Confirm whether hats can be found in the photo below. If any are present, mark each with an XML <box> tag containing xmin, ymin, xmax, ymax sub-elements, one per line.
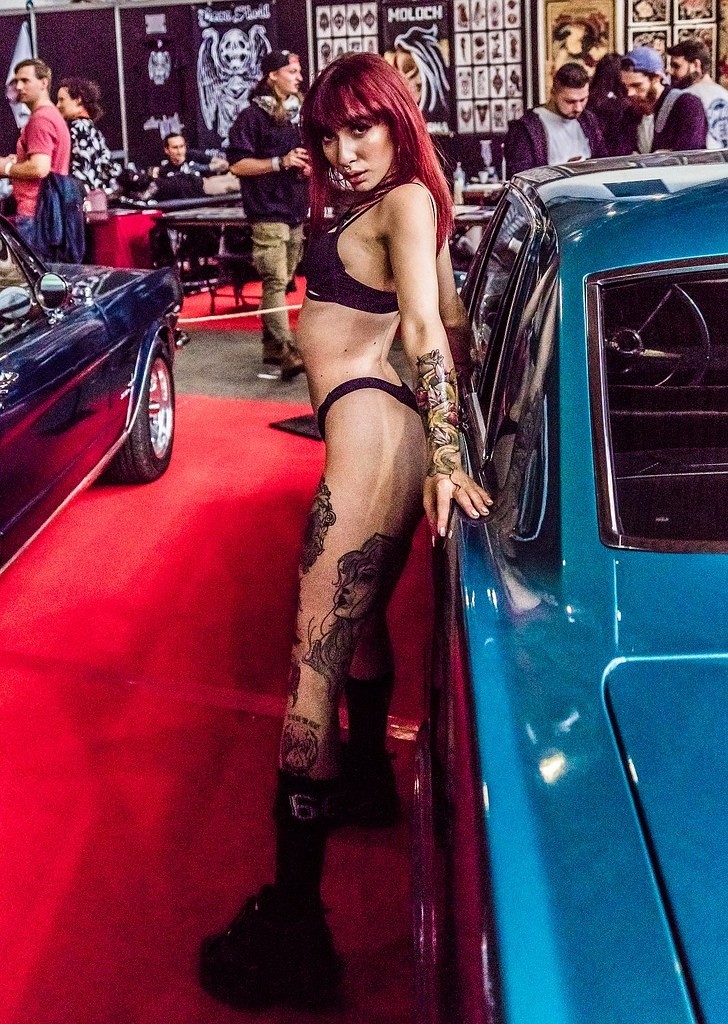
<box><xmin>261</xmin><ymin>49</ymin><xmax>299</xmax><ymax>72</ymax></box>
<box><xmin>621</xmin><ymin>46</ymin><xmax>668</xmax><ymax>80</ymax></box>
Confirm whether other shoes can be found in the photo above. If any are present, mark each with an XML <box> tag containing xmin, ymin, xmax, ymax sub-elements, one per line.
<box><xmin>262</xmin><ymin>336</ymin><xmax>283</xmax><ymax>365</ymax></box>
<box><xmin>282</xmin><ymin>349</ymin><xmax>305</xmax><ymax>382</ymax></box>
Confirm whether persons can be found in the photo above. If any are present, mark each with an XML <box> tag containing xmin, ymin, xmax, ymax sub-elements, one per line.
<box><xmin>196</xmin><ymin>48</ymin><xmax>497</xmax><ymax>1020</ymax></box>
<box><xmin>55</xmin><ymin>76</ymin><xmax>119</xmax><ymax>266</ymax></box>
<box><xmin>586</xmin><ymin>51</ymin><xmax>635</xmax><ymax>125</ymax></box>
<box><xmin>605</xmin><ymin>45</ymin><xmax>710</xmax><ymax>157</ymax></box>
<box><xmin>224</xmin><ymin>50</ymin><xmax>312</xmax><ymax>382</ymax></box>
<box><xmin>502</xmin><ymin>62</ymin><xmax>611</xmax><ymax>183</ymax></box>
<box><xmin>0</xmin><ymin>58</ymin><xmax>72</xmax><ymax>262</ymax></box>
<box><xmin>134</xmin><ymin>134</ymin><xmax>230</xmax><ymax>297</ymax></box>
<box><xmin>664</xmin><ymin>40</ymin><xmax>727</xmax><ymax>149</ymax></box>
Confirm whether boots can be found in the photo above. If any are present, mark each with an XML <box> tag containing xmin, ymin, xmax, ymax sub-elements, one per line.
<box><xmin>203</xmin><ymin>770</ymin><xmax>334</xmax><ymax>1000</ymax></box>
<box><xmin>326</xmin><ymin>679</ymin><xmax>399</xmax><ymax>826</ymax></box>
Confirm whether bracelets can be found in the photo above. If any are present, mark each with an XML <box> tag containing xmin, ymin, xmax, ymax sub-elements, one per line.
<box><xmin>271</xmin><ymin>156</ymin><xmax>280</xmax><ymax>172</ymax></box>
<box><xmin>279</xmin><ymin>157</ymin><xmax>287</xmax><ymax>171</ymax></box>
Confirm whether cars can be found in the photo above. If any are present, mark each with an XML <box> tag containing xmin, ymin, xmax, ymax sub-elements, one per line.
<box><xmin>419</xmin><ymin>151</ymin><xmax>725</xmax><ymax>1024</ymax></box>
<box><xmin>0</xmin><ymin>215</ymin><xmax>186</xmax><ymax>574</ymax></box>
